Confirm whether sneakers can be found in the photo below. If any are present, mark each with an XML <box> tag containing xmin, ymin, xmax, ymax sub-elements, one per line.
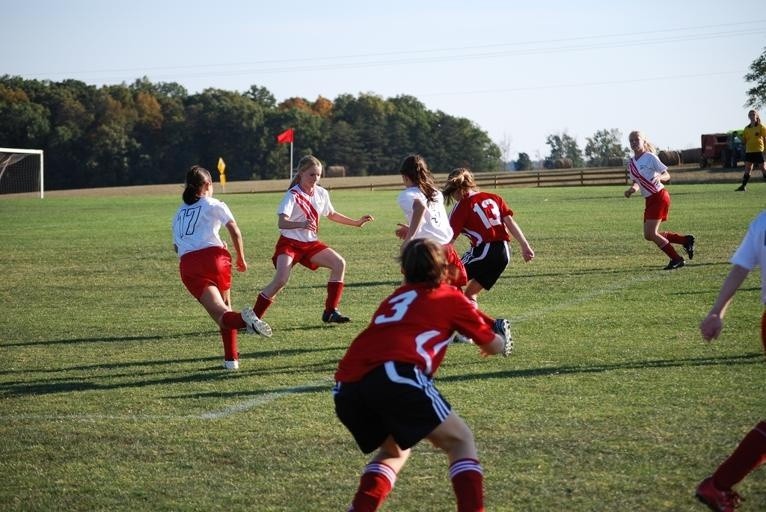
<box><xmin>223</xmin><ymin>359</ymin><xmax>240</xmax><ymax>371</ymax></box>
<box><xmin>320</xmin><ymin>308</ymin><xmax>353</xmax><ymax>324</ymax></box>
<box><xmin>240</xmin><ymin>306</ymin><xmax>273</xmax><ymax>339</ymax></box>
<box><xmin>663</xmin><ymin>257</ymin><xmax>685</xmax><ymax>270</ymax></box>
<box><xmin>682</xmin><ymin>234</ymin><xmax>695</xmax><ymax>261</ymax></box>
<box><xmin>692</xmin><ymin>476</ymin><xmax>744</xmax><ymax>512</ymax></box>
<box><xmin>492</xmin><ymin>317</ymin><xmax>515</xmax><ymax>358</ymax></box>
<box><xmin>733</xmin><ymin>184</ymin><xmax>747</xmax><ymax>193</ymax></box>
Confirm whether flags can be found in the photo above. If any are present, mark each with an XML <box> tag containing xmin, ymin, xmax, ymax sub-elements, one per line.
<box><xmin>278</xmin><ymin>129</ymin><xmax>293</xmax><ymax>143</ymax></box>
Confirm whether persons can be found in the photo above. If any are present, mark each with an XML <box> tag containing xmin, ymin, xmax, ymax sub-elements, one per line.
<box><xmin>734</xmin><ymin>110</ymin><xmax>766</xmax><ymax>191</ymax></box>
<box><xmin>395</xmin><ymin>155</ymin><xmax>513</xmax><ymax>357</ymax></box>
<box><xmin>331</xmin><ymin>238</ymin><xmax>504</xmax><ymax>512</ymax></box>
<box><xmin>172</xmin><ymin>165</ymin><xmax>272</xmax><ymax>370</ymax></box>
<box><xmin>625</xmin><ymin>131</ymin><xmax>695</xmax><ymax>270</ymax></box>
<box><xmin>438</xmin><ymin>167</ymin><xmax>535</xmax><ymax>343</ymax></box>
<box><xmin>699</xmin><ymin>211</ymin><xmax>766</xmax><ymax>511</ymax></box>
<box><xmin>246</xmin><ymin>155</ymin><xmax>375</xmax><ymax>333</ymax></box>
